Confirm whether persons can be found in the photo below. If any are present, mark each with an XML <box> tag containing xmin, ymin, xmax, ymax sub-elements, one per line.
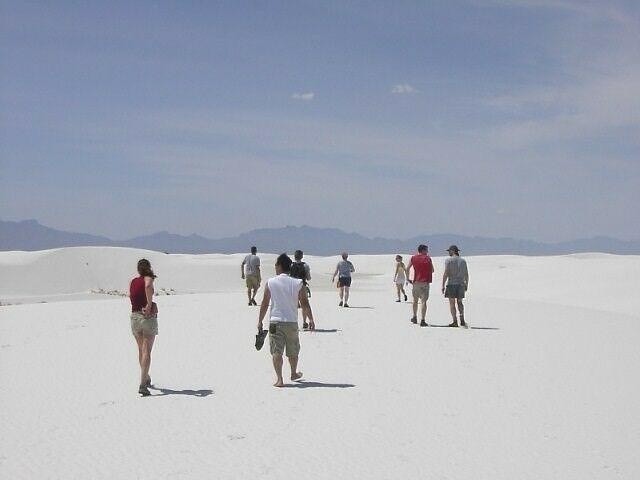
<box><xmin>258</xmin><ymin>255</ymin><xmax>315</xmax><ymax>386</ymax></box>
<box><xmin>290</xmin><ymin>250</ymin><xmax>311</xmax><ymax>329</ymax></box>
<box><xmin>405</xmin><ymin>245</ymin><xmax>434</xmax><ymax>327</ymax></box>
<box><xmin>241</xmin><ymin>246</ymin><xmax>262</xmax><ymax>306</ymax></box>
<box><xmin>393</xmin><ymin>255</ymin><xmax>407</xmax><ymax>302</ymax></box>
<box><xmin>442</xmin><ymin>245</ymin><xmax>469</xmax><ymax>328</ymax></box>
<box><xmin>333</xmin><ymin>253</ymin><xmax>354</xmax><ymax>307</ymax></box>
<box><xmin>129</xmin><ymin>259</ymin><xmax>159</xmax><ymax>395</ymax></box>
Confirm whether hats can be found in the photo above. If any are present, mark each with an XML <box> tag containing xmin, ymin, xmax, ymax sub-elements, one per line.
<box><xmin>446</xmin><ymin>245</ymin><xmax>458</xmax><ymax>251</ymax></box>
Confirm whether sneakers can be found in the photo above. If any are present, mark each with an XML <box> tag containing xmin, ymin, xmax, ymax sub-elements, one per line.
<box><xmin>449</xmin><ymin>320</ymin><xmax>465</xmax><ymax>326</ymax></box>
<box><xmin>339</xmin><ymin>301</ymin><xmax>349</xmax><ymax>307</ymax></box>
<box><xmin>411</xmin><ymin>316</ymin><xmax>428</xmax><ymax>326</ymax></box>
<box><xmin>139</xmin><ymin>380</ymin><xmax>150</xmax><ymax>395</ymax></box>
<box><xmin>396</xmin><ymin>295</ymin><xmax>407</xmax><ymax>302</ymax></box>
<box><xmin>255</xmin><ymin>328</ymin><xmax>267</xmax><ymax>350</ymax></box>
<box><xmin>303</xmin><ymin>323</ymin><xmax>308</xmax><ymax>328</ymax></box>
<box><xmin>249</xmin><ymin>298</ymin><xmax>256</xmax><ymax>306</ymax></box>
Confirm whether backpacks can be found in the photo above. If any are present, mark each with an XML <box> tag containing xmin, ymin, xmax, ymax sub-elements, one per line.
<box><xmin>290</xmin><ymin>262</ymin><xmax>305</xmax><ymax>286</ymax></box>
